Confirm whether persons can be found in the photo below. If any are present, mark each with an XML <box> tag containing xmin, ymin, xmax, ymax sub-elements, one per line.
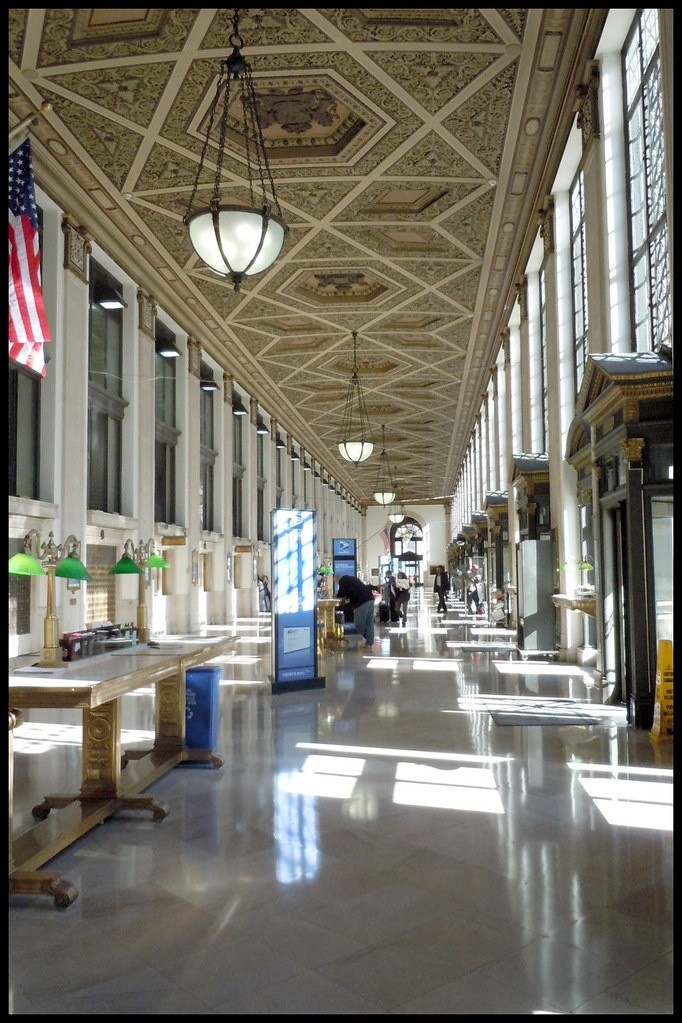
<box><xmin>334</xmin><ymin>574</ymin><xmax>375</xmax><ymax>648</ymax></box>
<box><xmin>318</xmin><ymin>573</ymin><xmax>325</xmax><ymax>598</ymax></box>
<box><xmin>257</xmin><ymin>574</ymin><xmax>271</xmax><ymax>612</ymax></box>
<box><xmin>434</xmin><ymin>565</ymin><xmax>450</xmax><ymax>613</ymax></box>
<box><xmin>469</xmin><ymin>565</ymin><xmax>479</xmax><ymax>614</ymax></box>
<box><xmin>377</xmin><ymin>570</ymin><xmax>410</xmax><ymax>625</ymax></box>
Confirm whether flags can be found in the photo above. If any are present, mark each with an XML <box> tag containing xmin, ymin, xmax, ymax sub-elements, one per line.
<box><xmin>8</xmin><ymin>136</ymin><xmax>52</xmax><ymax>380</ymax></box>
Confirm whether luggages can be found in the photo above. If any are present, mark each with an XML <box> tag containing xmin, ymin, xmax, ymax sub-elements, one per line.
<box><xmin>379</xmin><ymin>587</ymin><xmax>389</xmax><ymax>623</ymax></box>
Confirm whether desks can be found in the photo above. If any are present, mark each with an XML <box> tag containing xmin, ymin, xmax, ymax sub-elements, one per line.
<box><xmin>552</xmin><ymin>593</ymin><xmax>596</xmax><ymax>619</ymax></box>
<box><xmin>9</xmin><ymin>636</ymin><xmax>239</xmax><ymax>908</ymax></box>
<box><xmin>317</xmin><ymin>597</ymin><xmax>351</xmax><ymax>679</ymax></box>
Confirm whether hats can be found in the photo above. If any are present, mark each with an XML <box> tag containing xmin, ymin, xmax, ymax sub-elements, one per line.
<box><xmin>472</xmin><ymin>564</ymin><xmax>478</xmax><ymax>570</ymax></box>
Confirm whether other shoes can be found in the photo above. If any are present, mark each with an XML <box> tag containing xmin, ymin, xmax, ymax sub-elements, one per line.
<box><xmin>359</xmin><ymin>644</ymin><xmax>373</xmax><ymax>648</ymax></box>
<box><xmin>402</xmin><ymin>622</ymin><xmax>405</xmax><ymax>627</ymax></box>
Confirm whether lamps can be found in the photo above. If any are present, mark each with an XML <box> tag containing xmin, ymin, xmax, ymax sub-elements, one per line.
<box><xmin>155</xmin><ymin>336</ymin><xmax>182</xmax><ymax>360</ymax></box>
<box><xmin>201</xmin><ymin>374</ymin><xmax>220</xmax><ymax>393</ymax></box>
<box><xmin>106</xmin><ymin>537</ymin><xmax>172</xmax><ymax>645</ymax></box>
<box><xmin>317</xmin><ymin>561</ymin><xmax>334</xmax><ymax>600</ymax></box>
<box><xmin>574</xmin><ymin>555</ymin><xmax>596</xmax><ymax>598</ymax></box>
<box><xmin>181</xmin><ymin>9</ymin><xmax>290</xmax><ymax>293</ymax></box>
<box><xmin>373</xmin><ymin>424</ymin><xmax>397</xmax><ymax>510</ymax></box>
<box><xmin>397</xmin><ymin>504</ymin><xmax>413</xmax><ymax>542</ymax></box>
<box><xmin>336</xmin><ymin>331</ymin><xmax>376</xmax><ymax>469</ymax></box>
<box><xmin>8</xmin><ymin>530</ymin><xmax>94</xmax><ymax>670</ymax></box>
<box><xmin>386</xmin><ymin>486</ymin><xmax>405</xmax><ymax>524</ymax></box>
<box><xmin>233</xmin><ymin>399</ymin><xmax>368</xmax><ymax>519</ymax></box>
<box><xmin>93</xmin><ymin>273</ymin><xmax>127</xmax><ymax>311</ymax></box>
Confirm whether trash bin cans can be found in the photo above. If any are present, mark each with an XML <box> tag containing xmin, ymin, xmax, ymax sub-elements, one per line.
<box><xmin>186</xmin><ymin>665</ymin><xmax>223</xmax><ymax>750</ymax></box>
